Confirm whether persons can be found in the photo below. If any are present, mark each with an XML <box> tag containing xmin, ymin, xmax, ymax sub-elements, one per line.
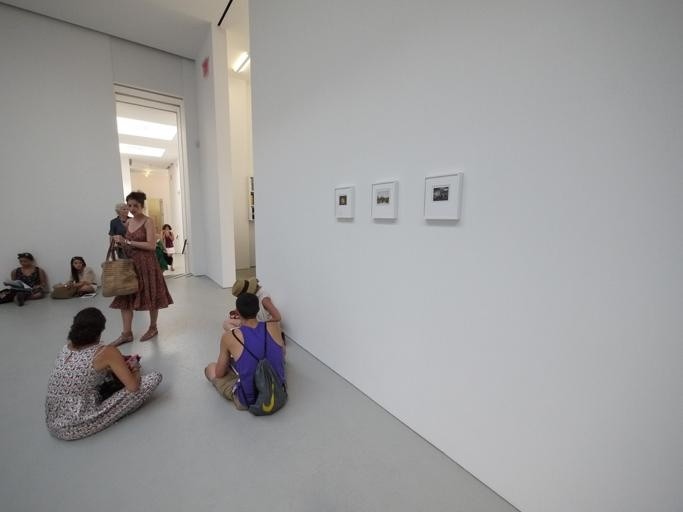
<box><xmin>204</xmin><ymin>293</ymin><xmax>287</xmax><ymax>411</ymax></box>
<box><xmin>222</xmin><ymin>277</ymin><xmax>281</xmax><ymax>332</ymax></box>
<box><xmin>66</xmin><ymin>256</ymin><xmax>97</xmax><ymax>296</ymax></box>
<box><xmin>161</xmin><ymin>224</ymin><xmax>174</xmax><ymax>271</ymax></box>
<box><xmin>109</xmin><ymin>203</ymin><xmax>133</xmax><ymax>257</ymax></box>
<box><xmin>0</xmin><ymin>253</ymin><xmax>48</xmax><ymax>306</ymax></box>
<box><xmin>45</xmin><ymin>307</ymin><xmax>163</xmax><ymax>442</ymax></box>
<box><xmin>106</xmin><ymin>190</ymin><xmax>174</xmax><ymax>348</ymax></box>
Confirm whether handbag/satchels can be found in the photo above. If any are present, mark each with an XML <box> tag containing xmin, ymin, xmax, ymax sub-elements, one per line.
<box><xmin>51</xmin><ymin>283</ymin><xmax>78</xmax><ymax>298</ymax></box>
<box><xmin>101</xmin><ymin>238</ymin><xmax>139</xmax><ymax>297</ymax></box>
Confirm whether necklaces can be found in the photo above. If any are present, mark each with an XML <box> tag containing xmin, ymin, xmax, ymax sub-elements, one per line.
<box><xmin>120</xmin><ymin>218</ymin><xmax>128</xmax><ymax>223</ymax></box>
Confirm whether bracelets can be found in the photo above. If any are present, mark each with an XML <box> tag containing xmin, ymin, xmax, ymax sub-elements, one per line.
<box><xmin>127</xmin><ymin>240</ymin><xmax>131</xmax><ymax>246</ymax></box>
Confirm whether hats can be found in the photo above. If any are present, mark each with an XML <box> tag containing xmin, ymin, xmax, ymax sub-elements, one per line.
<box><xmin>232</xmin><ymin>277</ymin><xmax>258</xmax><ymax>297</ymax></box>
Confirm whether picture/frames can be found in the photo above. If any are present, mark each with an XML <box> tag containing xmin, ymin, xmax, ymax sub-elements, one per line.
<box><xmin>424</xmin><ymin>172</ymin><xmax>463</xmax><ymax>220</ymax></box>
<box><xmin>371</xmin><ymin>181</ymin><xmax>397</xmax><ymax>219</ymax></box>
<box><xmin>334</xmin><ymin>186</ymin><xmax>355</xmax><ymax>218</ymax></box>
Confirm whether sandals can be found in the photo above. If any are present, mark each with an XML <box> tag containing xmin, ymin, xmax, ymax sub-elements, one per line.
<box><xmin>140</xmin><ymin>326</ymin><xmax>158</xmax><ymax>341</ymax></box>
<box><xmin>112</xmin><ymin>332</ymin><xmax>133</xmax><ymax>346</ymax></box>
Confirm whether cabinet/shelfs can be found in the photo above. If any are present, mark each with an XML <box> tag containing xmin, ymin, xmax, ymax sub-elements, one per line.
<box><xmin>247</xmin><ymin>177</ymin><xmax>256</xmax><ymax>267</ymax></box>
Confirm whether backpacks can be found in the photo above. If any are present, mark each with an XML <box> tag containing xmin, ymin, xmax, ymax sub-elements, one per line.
<box><xmin>232</xmin><ymin>322</ymin><xmax>287</xmax><ymax>415</ymax></box>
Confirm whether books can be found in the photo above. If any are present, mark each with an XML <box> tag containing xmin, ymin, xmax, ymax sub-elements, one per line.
<box><xmin>2</xmin><ymin>279</ymin><xmax>32</xmax><ymax>290</ymax></box>
